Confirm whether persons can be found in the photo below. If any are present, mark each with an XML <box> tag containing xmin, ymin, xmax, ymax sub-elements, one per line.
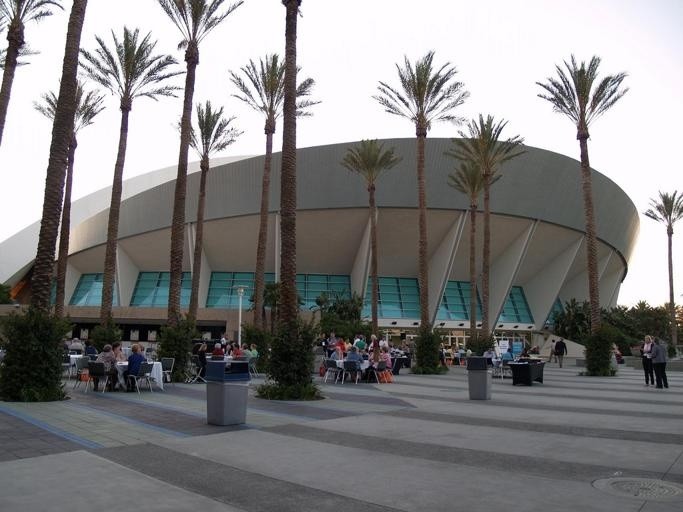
<box><xmin>554</xmin><ymin>337</ymin><xmax>567</xmax><ymax>368</ymax></box>
<box><xmin>646</xmin><ymin>337</ymin><xmax>668</xmax><ymax>388</ymax></box>
<box><xmin>190</xmin><ymin>335</ymin><xmax>257</xmax><ymax>382</ymax></box>
<box><xmin>481</xmin><ymin>347</ymin><xmax>496</xmax><ymax>364</ymax></box>
<box><xmin>501</xmin><ymin>348</ymin><xmax>515</xmax><ymax>362</ymax></box>
<box><xmin>640</xmin><ymin>335</ymin><xmax>654</xmax><ymax>385</ymax></box>
<box><xmin>314</xmin><ymin>328</ymin><xmax>472</xmax><ymax>384</ymax></box>
<box><xmin>546</xmin><ymin>339</ymin><xmax>557</xmax><ymax>363</ymax></box>
<box><xmin>60</xmin><ymin>337</ymin><xmax>147</xmax><ymax>392</ymax></box>
<box><xmin>517</xmin><ymin>345</ymin><xmax>540</xmax><ymax>363</ymax></box>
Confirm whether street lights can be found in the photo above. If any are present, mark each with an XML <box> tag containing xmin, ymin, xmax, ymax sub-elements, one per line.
<box><xmin>231</xmin><ymin>285</ymin><xmax>248</xmax><ymax>348</ymax></box>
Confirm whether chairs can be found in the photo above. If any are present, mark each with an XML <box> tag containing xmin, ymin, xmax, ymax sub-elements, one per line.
<box><xmin>59</xmin><ymin>343</ymin><xmax>175</xmax><ymax>394</ymax></box>
<box><xmin>442</xmin><ymin>350</ymin><xmax>538</xmax><ymax>384</ymax></box>
<box><xmin>183</xmin><ymin>351</ymin><xmax>260</xmax><ymax>384</ymax></box>
<box><xmin>321</xmin><ymin>352</ymin><xmax>413</xmax><ymax>386</ymax></box>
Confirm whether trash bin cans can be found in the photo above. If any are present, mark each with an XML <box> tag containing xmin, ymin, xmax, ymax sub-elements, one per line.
<box><xmin>466</xmin><ymin>356</ymin><xmax>493</xmax><ymax>399</ymax></box>
<box><xmin>206</xmin><ymin>361</ymin><xmax>252</xmax><ymax>426</ymax></box>
